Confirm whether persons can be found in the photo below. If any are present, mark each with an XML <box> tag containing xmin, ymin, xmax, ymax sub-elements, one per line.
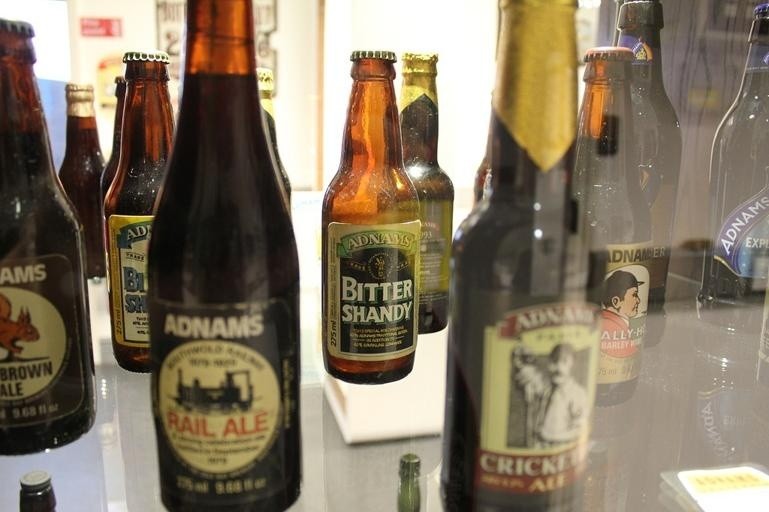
<box><xmin>598</xmin><ymin>270</ymin><xmax>646</xmax><ymax>339</ymax></box>
<box><xmin>514</xmin><ymin>343</ymin><xmax>586</xmax><ymax>450</ymax></box>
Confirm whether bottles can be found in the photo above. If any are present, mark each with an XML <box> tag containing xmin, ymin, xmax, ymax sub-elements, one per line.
<box><xmin>390</xmin><ymin>51</ymin><xmax>455</xmax><ymax>338</ymax></box>
<box><xmin>18</xmin><ymin>470</ymin><xmax>58</xmax><ymax>512</ymax></box>
<box><xmin>396</xmin><ymin>453</ymin><xmax>422</xmax><ymax>511</ymax></box>
<box><xmin>611</xmin><ymin>1</ymin><xmax>686</xmax><ymax>348</ymax></box>
<box><xmin>435</xmin><ymin>1</ymin><xmax>604</xmax><ymax>511</ymax></box>
<box><xmin>315</xmin><ymin>50</ymin><xmax>424</xmax><ymax>390</ymax></box>
<box><xmin>144</xmin><ymin>2</ymin><xmax>300</xmax><ymax>511</ymax></box>
<box><xmin>98</xmin><ymin>42</ymin><xmax>178</xmax><ymax>379</ymax></box>
<box><xmin>54</xmin><ymin>82</ymin><xmax>111</xmax><ymax>286</ymax></box>
<box><xmin>695</xmin><ymin>4</ymin><xmax>769</xmax><ymax>334</ymax></box>
<box><xmin>0</xmin><ymin>16</ymin><xmax>96</xmax><ymax>459</ymax></box>
<box><xmin>575</xmin><ymin>48</ymin><xmax>646</xmax><ymax>404</ymax></box>
